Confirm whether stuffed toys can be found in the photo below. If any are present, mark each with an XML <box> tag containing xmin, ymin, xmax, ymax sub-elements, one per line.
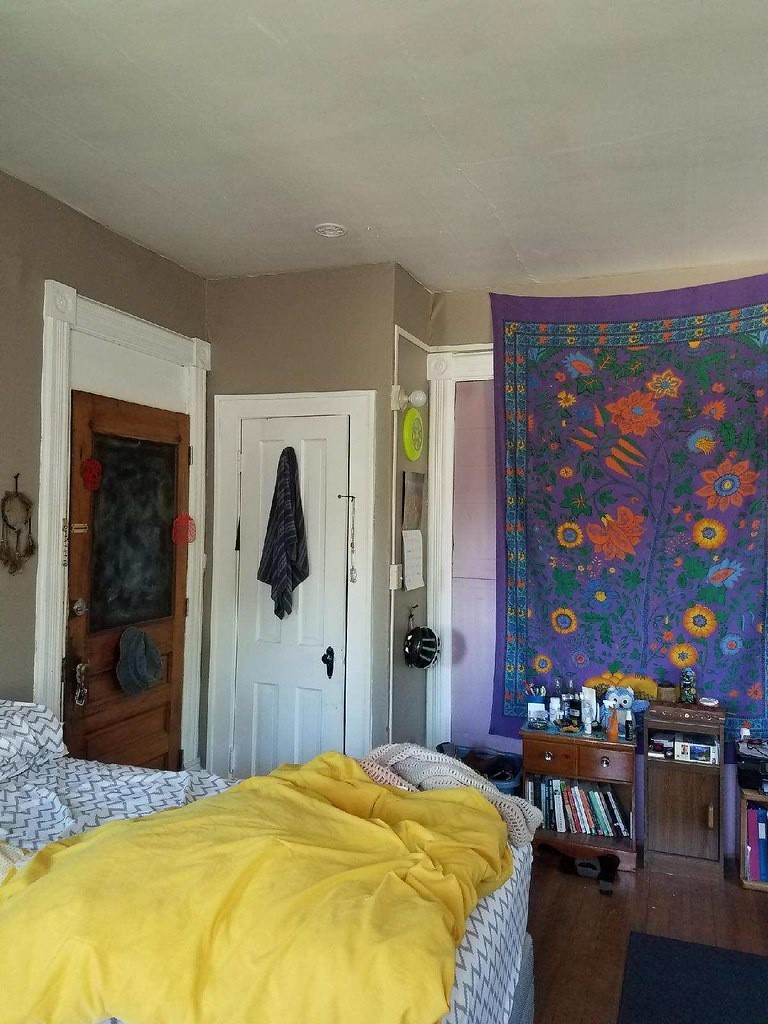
<box><xmin>598</xmin><ymin>681</ymin><xmax>650</xmax><ymax>738</ymax></box>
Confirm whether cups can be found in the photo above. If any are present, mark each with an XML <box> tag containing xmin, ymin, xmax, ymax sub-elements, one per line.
<box><xmin>526</xmin><ymin>695</ymin><xmax>545</xmax><ymax>707</ymax></box>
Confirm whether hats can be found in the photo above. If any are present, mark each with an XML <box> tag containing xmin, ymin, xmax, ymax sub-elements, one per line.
<box><xmin>116</xmin><ymin>626</ymin><xmax>162</xmax><ymax>696</ymax></box>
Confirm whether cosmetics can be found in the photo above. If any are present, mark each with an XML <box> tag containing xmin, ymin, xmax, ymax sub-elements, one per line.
<box><xmin>520</xmin><ymin>679</ymin><xmax>633</xmax><ymax>743</ymax></box>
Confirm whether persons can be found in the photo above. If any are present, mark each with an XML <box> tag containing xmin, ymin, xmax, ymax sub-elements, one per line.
<box><xmin>680</xmin><ymin>744</ymin><xmax>688</xmax><ymax>754</ymax></box>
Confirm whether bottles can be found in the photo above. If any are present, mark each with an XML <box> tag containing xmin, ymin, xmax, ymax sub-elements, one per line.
<box><xmin>625</xmin><ymin>710</ymin><xmax>633</xmax><ymax>741</ymax></box>
<box><xmin>528</xmin><ymin>710</ymin><xmax>548</xmax><ymax>730</ymax></box>
<box><xmin>548</xmin><ymin>677</ymin><xmax>585</xmax><ymax>727</ymax></box>
<box><xmin>680</xmin><ymin>667</ymin><xmax>696</xmax><ymax>703</ymax></box>
<box><xmin>658</xmin><ymin>682</ymin><xmax>676</xmax><ymax>702</ymax></box>
<box><xmin>739</xmin><ymin>728</ymin><xmax>751</xmax><ymax>740</ymax></box>
<box><xmin>584</xmin><ymin>717</ymin><xmax>592</xmax><ymax>734</ymax></box>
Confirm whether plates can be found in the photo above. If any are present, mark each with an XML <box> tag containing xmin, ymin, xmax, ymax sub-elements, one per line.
<box><xmin>700</xmin><ymin>696</ymin><xmax>719</xmax><ymax>707</ymax></box>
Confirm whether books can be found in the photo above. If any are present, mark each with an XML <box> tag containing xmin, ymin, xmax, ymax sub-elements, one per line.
<box><xmin>746</xmin><ymin>808</ymin><xmax>767</xmax><ymax>883</ymax></box>
<box><xmin>524</xmin><ymin>774</ymin><xmax>629</xmax><ymax>838</ymax></box>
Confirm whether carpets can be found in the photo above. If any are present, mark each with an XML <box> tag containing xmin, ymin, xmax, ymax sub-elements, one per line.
<box><xmin>616</xmin><ymin>931</ymin><xmax>768</xmax><ymax>1024</ymax></box>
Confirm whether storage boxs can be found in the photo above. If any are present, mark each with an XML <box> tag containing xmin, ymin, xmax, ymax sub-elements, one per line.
<box><xmin>435</xmin><ymin>741</ymin><xmax>523</xmax><ymax>795</ymax></box>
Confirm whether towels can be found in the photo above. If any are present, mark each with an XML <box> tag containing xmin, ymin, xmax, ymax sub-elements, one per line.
<box><xmin>256</xmin><ymin>446</ymin><xmax>308</xmax><ymax>621</ymax></box>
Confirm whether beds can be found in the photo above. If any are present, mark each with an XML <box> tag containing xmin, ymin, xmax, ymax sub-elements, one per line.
<box><xmin>0</xmin><ymin>758</ymin><xmax>539</xmax><ymax>1024</ymax></box>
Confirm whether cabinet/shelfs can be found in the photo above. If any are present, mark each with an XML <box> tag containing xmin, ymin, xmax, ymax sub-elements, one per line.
<box><xmin>517</xmin><ymin>723</ymin><xmax>639</xmax><ymax>873</ymax></box>
<box><xmin>643</xmin><ymin>707</ymin><xmax>725</xmax><ymax>881</ymax></box>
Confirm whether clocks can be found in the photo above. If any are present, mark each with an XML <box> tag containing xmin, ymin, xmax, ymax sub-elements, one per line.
<box><xmin>402</xmin><ymin>408</ymin><xmax>424</xmax><ymax>462</ymax></box>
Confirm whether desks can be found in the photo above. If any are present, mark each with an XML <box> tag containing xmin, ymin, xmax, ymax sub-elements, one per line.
<box><xmin>736</xmin><ymin>788</ymin><xmax>768</xmax><ymax>893</ymax></box>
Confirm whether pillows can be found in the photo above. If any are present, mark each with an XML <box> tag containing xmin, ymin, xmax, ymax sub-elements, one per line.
<box><xmin>0</xmin><ymin>699</ymin><xmax>70</xmax><ymax>782</ymax></box>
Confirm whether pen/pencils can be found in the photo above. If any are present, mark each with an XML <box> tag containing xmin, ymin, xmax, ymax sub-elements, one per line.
<box><xmin>522</xmin><ymin>680</ymin><xmax>547</xmax><ymax>697</ymax></box>
